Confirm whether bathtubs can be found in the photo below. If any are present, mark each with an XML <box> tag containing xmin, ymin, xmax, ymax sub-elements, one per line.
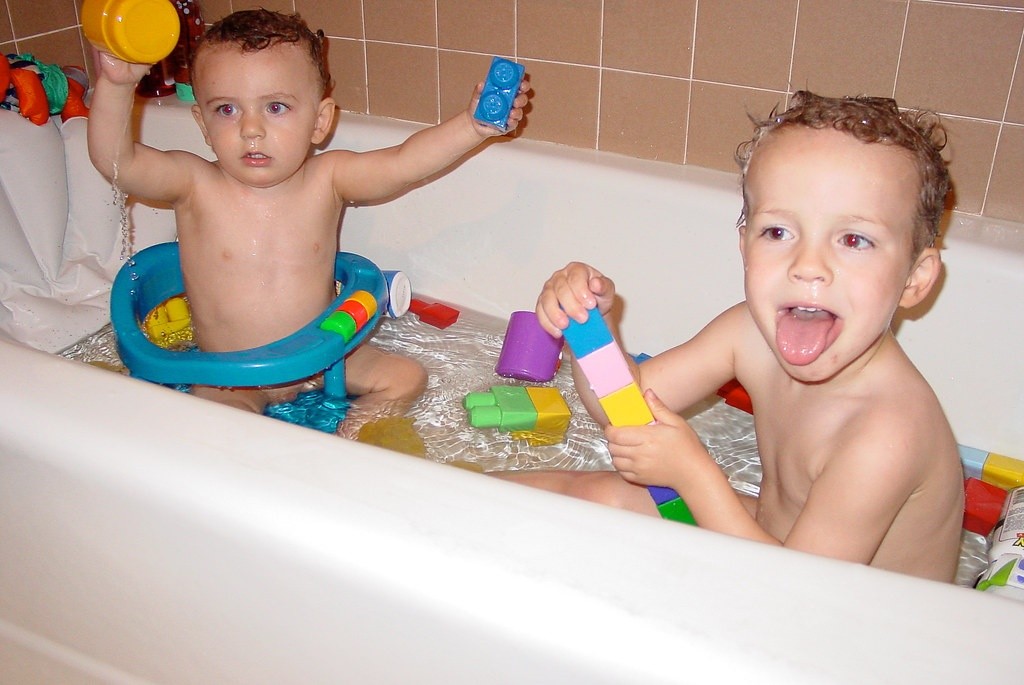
<box><xmin>0</xmin><ymin>88</ymin><xmax>1024</xmax><ymax>683</ymax></box>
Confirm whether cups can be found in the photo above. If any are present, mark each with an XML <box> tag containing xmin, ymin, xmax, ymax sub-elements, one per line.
<box><xmin>81</xmin><ymin>0</ymin><xmax>180</xmax><ymax>64</ymax></box>
<box><xmin>334</xmin><ymin>270</ymin><xmax>411</xmax><ymax>320</ymax></box>
<box><xmin>495</xmin><ymin>311</ymin><xmax>564</xmax><ymax>382</ymax></box>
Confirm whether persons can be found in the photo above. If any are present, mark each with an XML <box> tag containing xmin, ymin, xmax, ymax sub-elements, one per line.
<box><xmin>485</xmin><ymin>90</ymin><xmax>965</xmax><ymax>585</ymax></box>
<box><xmin>86</xmin><ymin>10</ymin><xmax>531</xmax><ymax>417</ymax></box>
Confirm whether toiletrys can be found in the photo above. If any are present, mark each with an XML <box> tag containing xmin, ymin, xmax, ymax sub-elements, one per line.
<box><xmin>135</xmin><ymin>1</ymin><xmax>207</xmax><ymax>102</ymax></box>
